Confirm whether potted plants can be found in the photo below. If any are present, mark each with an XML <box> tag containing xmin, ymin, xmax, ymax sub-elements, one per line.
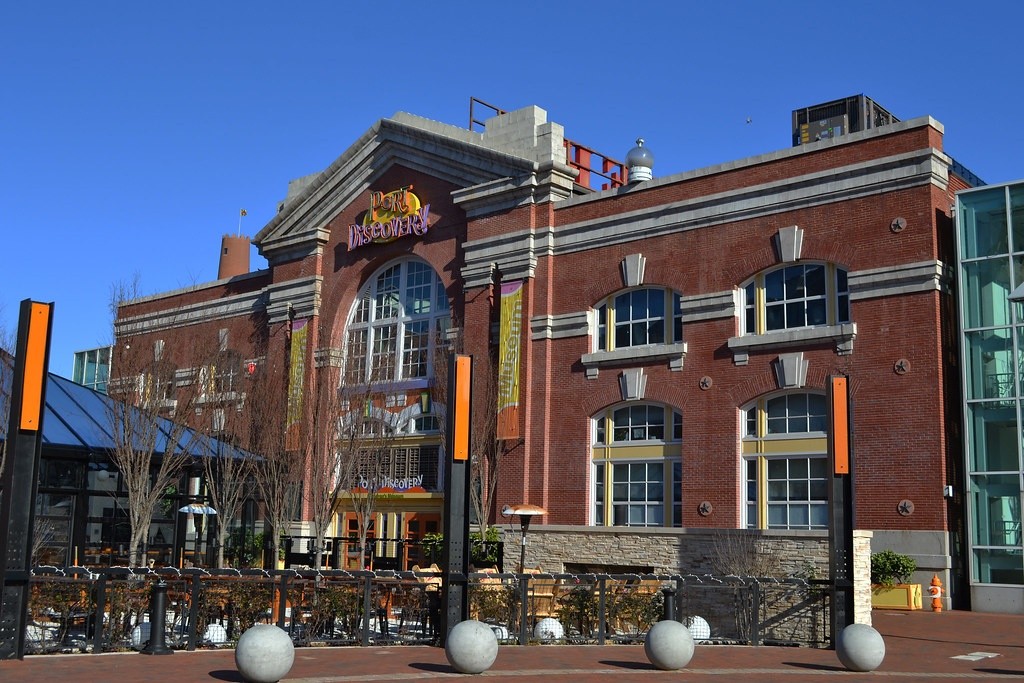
<box><xmin>871</xmin><ymin>548</ymin><xmax>923</xmax><ymax>611</ymax></box>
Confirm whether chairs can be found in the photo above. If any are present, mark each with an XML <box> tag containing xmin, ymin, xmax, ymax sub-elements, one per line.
<box><xmin>27</xmin><ymin>545</ymin><xmax>673</xmax><ymax>648</ymax></box>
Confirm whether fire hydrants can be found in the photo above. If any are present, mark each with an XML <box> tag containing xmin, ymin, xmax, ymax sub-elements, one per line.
<box><xmin>926</xmin><ymin>574</ymin><xmax>945</xmax><ymax>613</ymax></box>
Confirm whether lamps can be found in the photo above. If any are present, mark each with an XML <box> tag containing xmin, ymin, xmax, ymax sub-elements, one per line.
<box><xmin>98</xmin><ymin>470</ymin><xmax>119</xmax><ymax>483</ymax></box>
